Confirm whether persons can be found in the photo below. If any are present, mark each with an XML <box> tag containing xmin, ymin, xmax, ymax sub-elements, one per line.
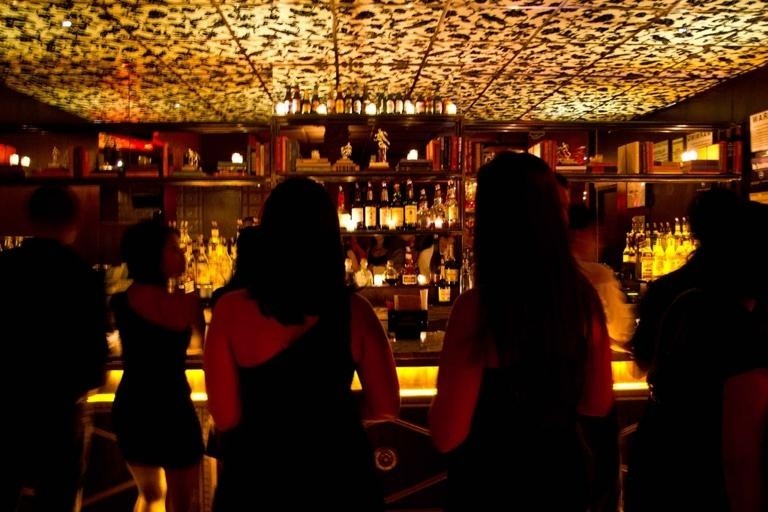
<box><xmin>0</xmin><ymin>180</ymin><xmax>110</xmax><ymax>510</ymax></box>
<box><xmin>422</xmin><ymin>148</ymin><xmax>618</xmax><ymax>511</ymax></box>
<box><xmin>549</xmin><ymin>170</ymin><xmax>573</xmax><ymax>230</ymax></box>
<box><xmin>102</xmin><ymin>213</ymin><xmax>206</xmax><ymax>509</ymax></box>
<box><xmin>210</xmin><ymin>224</ymin><xmax>262</xmax><ymax>310</ymax></box>
<box><xmin>200</xmin><ymin>172</ymin><xmax>404</xmax><ymax>510</ymax></box>
<box><xmin>626</xmin><ymin>185</ymin><xmax>768</xmax><ymax>509</ymax></box>
<box><xmin>561</xmin><ymin>200</ymin><xmax>638</xmax><ymax>350</ymax></box>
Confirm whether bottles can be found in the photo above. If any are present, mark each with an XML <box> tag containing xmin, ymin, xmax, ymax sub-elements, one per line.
<box><xmin>621</xmin><ymin>215</ymin><xmax>700</xmax><ymax>294</ymax></box>
<box><xmin>280</xmin><ymin>87</ymin><xmax>460</xmax><ymax>117</ymax></box>
<box><xmin>333</xmin><ymin>179</ymin><xmax>462</xmax><ymax>313</ymax></box>
<box><xmin>102</xmin><ymin>216</ymin><xmax>260</xmax><ymax>298</ymax></box>
